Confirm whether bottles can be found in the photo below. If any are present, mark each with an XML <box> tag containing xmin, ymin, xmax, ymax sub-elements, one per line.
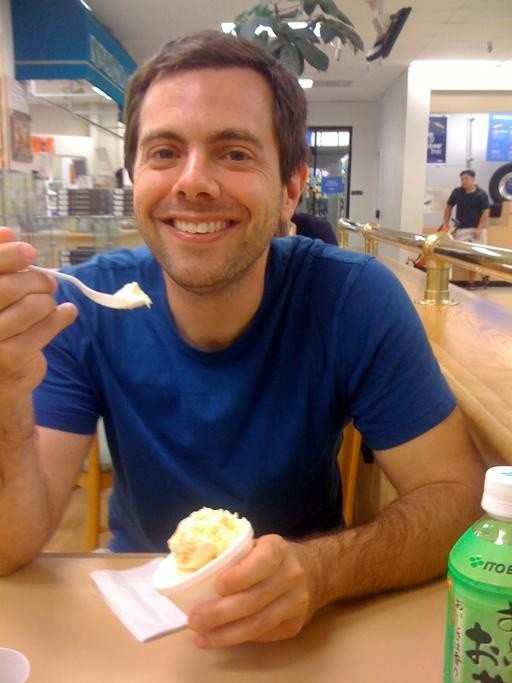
<box><xmin>445</xmin><ymin>465</ymin><xmax>511</xmax><ymax>682</ymax></box>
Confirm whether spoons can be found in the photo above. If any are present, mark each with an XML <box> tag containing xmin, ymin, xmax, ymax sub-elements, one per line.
<box><xmin>25</xmin><ymin>264</ymin><xmax>147</xmax><ymax>311</ymax></box>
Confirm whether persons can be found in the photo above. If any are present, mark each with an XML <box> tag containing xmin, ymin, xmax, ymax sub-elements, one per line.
<box><xmin>287</xmin><ymin>191</ymin><xmax>338</xmax><ymax>246</ymax></box>
<box><xmin>443</xmin><ymin>167</ymin><xmax>492</xmax><ymax>291</ymax></box>
<box><xmin>1</xmin><ymin>27</ymin><xmax>496</xmax><ymax>652</ymax></box>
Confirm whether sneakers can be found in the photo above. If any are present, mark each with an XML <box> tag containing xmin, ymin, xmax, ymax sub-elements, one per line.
<box><xmin>466</xmin><ymin>273</ymin><xmax>490</xmax><ymax>293</ymax></box>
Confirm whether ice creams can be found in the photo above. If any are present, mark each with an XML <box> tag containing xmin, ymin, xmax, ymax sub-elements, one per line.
<box><xmin>167</xmin><ymin>504</ymin><xmax>246</xmax><ymax>572</ymax></box>
<box><xmin>113</xmin><ymin>279</ymin><xmax>153</xmax><ymax>313</ymax></box>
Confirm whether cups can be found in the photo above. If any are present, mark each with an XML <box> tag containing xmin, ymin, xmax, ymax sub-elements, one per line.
<box><xmin>154</xmin><ymin>516</ymin><xmax>255</xmax><ymax>621</ymax></box>
